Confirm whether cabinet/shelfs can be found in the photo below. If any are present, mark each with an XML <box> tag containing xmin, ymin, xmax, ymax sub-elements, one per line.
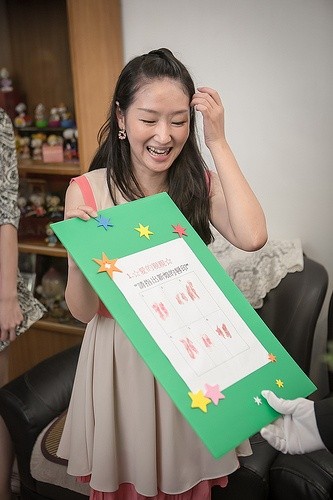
<box><xmin>1</xmin><ymin>0</ymin><xmax>125</xmax><ymax>385</ymax></box>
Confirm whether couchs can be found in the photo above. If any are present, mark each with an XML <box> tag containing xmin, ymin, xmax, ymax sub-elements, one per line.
<box><xmin>0</xmin><ymin>251</ymin><xmax>328</xmax><ymax>500</ymax></box>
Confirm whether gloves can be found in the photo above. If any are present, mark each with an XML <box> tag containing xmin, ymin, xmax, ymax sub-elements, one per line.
<box><xmin>259</xmin><ymin>389</ymin><xmax>327</xmax><ymax>456</ymax></box>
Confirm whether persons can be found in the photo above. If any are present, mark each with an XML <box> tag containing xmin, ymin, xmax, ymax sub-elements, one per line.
<box><xmin>260</xmin><ymin>390</ymin><xmax>333</xmax><ymax>455</ymax></box>
<box><xmin>56</xmin><ymin>47</ymin><xmax>268</xmax><ymax>500</ymax></box>
<box><xmin>0</xmin><ymin>105</ymin><xmax>50</xmax><ymax>500</ymax></box>
